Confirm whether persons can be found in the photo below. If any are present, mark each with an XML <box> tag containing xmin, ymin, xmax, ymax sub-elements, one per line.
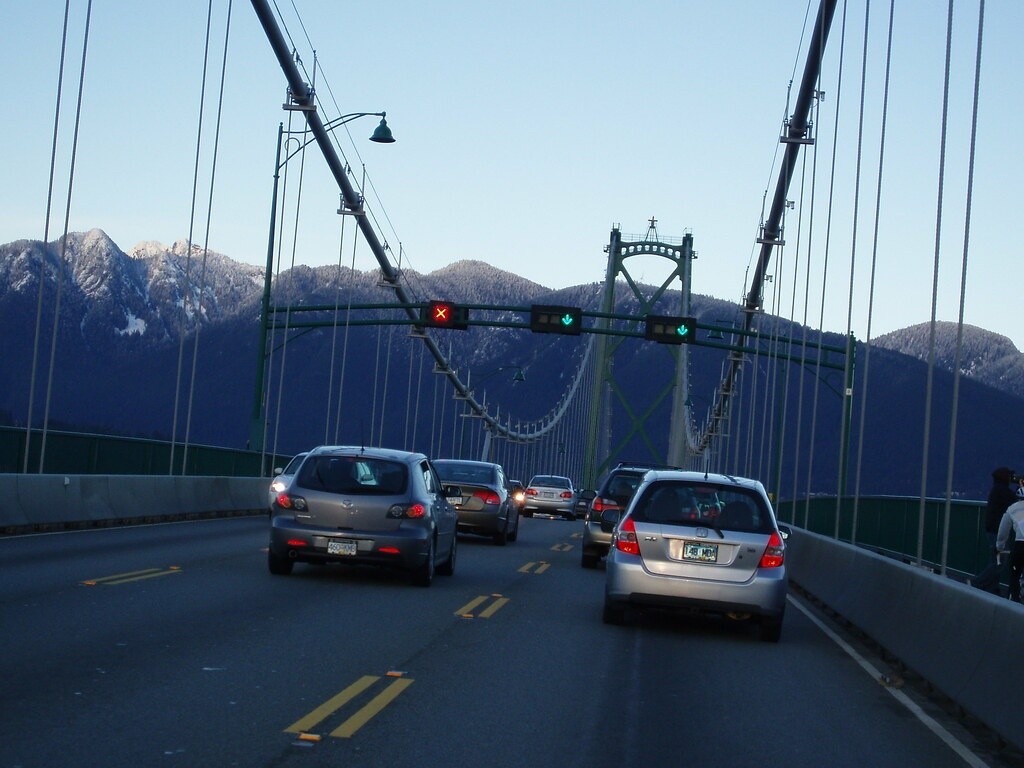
<box><xmin>995</xmin><ymin>485</ymin><xmax>1024</xmax><ymax>603</ymax></box>
<box><xmin>973</xmin><ymin>467</ymin><xmax>1020</xmax><ymax>594</ymax></box>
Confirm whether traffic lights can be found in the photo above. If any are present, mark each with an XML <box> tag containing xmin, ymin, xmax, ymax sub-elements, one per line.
<box><xmin>645</xmin><ymin>315</ymin><xmax>695</xmax><ymax>343</ymax></box>
<box><xmin>428</xmin><ymin>300</ymin><xmax>454</xmax><ymax>325</ymax></box>
<box><xmin>531</xmin><ymin>305</ymin><xmax>581</xmax><ymax>334</ymax></box>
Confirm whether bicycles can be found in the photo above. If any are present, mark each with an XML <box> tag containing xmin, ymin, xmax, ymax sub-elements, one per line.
<box><xmin>993</xmin><ymin>546</ymin><xmax>1024</xmax><ymax>604</ymax></box>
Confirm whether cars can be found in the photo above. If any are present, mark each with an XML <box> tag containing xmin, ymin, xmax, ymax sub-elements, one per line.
<box><xmin>525</xmin><ymin>474</ymin><xmax>577</xmax><ymax>519</ymax></box>
<box><xmin>573</xmin><ymin>489</ymin><xmax>600</xmax><ymax>518</ymax></box>
<box><xmin>265</xmin><ymin>420</ymin><xmax>458</xmax><ymax>581</ymax></box>
<box><xmin>506</xmin><ymin>478</ymin><xmax>527</xmax><ymax>509</ymax></box>
<box><xmin>265</xmin><ymin>450</ymin><xmax>313</xmax><ymax>514</ymax></box>
<box><xmin>600</xmin><ymin>456</ymin><xmax>793</xmax><ymax>639</ymax></box>
<box><xmin>425</xmin><ymin>457</ymin><xmax>518</xmax><ymax>543</ymax></box>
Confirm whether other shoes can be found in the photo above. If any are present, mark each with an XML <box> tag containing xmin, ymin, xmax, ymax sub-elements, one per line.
<box><xmin>1009</xmin><ymin>595</ymin><xmax>1020</xmax><ymax>602</ymax></box>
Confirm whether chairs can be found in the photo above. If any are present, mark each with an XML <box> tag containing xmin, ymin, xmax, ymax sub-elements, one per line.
<box><xmin>378</xmin><ymin>472</ymin><xmax>402</xmax><ymax>495</ymax></box>
<box><xmin>322</xmin><ymin>461</ymin><xmax>360</xmax><ymax>492</ymax></box>
<box><xmin>648</xmin><ymin>488</ymin><xmax>685</xmax><ymax>522</ymax></box>
<box><xmin>718</xmin><ymin>500</ymin><xmax>755</xmax><ymax>531</ymax></box>
<box><xmin>613</xmin><ymin>482</ymin><xmax>637</xmax><ymax>500</ymax></box>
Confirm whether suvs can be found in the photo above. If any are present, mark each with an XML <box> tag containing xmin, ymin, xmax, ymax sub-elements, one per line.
<box><xmin>579</xmin><ymin>461</ymin><xmax>684</xmax><ymax>568</ymax></box>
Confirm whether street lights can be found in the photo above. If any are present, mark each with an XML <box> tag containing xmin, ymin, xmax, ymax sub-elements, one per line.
<box><xmin>250</xmin><ymin>108</ymin><xmax>398</xmax><ymax>445</ymax></box>
